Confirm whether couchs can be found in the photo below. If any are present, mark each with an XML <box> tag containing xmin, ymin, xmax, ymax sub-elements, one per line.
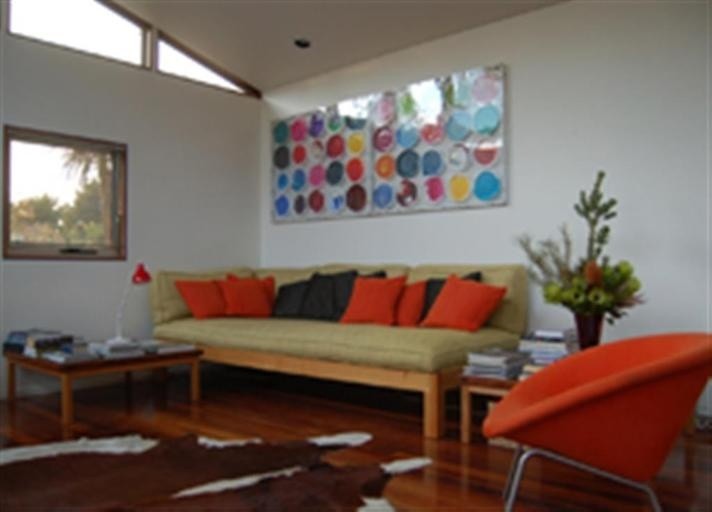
<box><xmin>151</xmin><ymin>262</ymin><xmax>529</xmax><ymax>438</ymax></box>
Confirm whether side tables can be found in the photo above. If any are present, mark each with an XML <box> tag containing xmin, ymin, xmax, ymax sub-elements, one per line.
<box><xmin>459</xmin><ymin>377</ymin><xmax>512</xmax><ymax>445</ymax></box>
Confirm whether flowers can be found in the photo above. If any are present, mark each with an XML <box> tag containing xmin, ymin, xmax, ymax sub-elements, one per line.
<box><xmin>517</xmin><ymin>168</ymin><xmax>646</xmax><ymax>348</ymax></box>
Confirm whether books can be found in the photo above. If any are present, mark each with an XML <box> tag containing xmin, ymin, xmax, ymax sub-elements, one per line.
<box><xmin>134</xmin><ymin>339</ymin><xmax>201</xmax><ymax>355</ymax></box>
<box><xmin>463</xmin><ymin>346</ymin><xmax>521</xmax><ymax>381</ymax></box>
<box><xmin>519</xmin><ymin>327</ymin><xmax>579</xmax><ymax>383</ymax></box>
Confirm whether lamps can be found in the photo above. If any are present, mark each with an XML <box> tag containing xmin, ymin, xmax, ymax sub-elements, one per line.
<box><xmin>108</xmin><ymin>263</ymin><xmax>151</xmax><ymax>346</ymax></box>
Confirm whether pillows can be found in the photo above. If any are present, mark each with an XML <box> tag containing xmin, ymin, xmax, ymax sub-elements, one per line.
<box><xmin>214</xmin><ymin>279</ymin><xmax>270</xmax><ymax>316</ymax></box>
<box><xmin>421</xmin><ymin>270</ymin><xmax>482</xmax><ymax>320</ymax></box>
<box><xmin>420</xmin><ymin>273</ymin><xmax>508</xmax><ymax>331</ymax></box>
<box><xmin>300</xmin><ymin>269</ymin><xmax>357</xmax><ymax>317</ymax></box>
<box><xmin>227</xmin><ymin>272</ymin><xmax>275</xmax><ymax>317</ymax></box>
<box><xmin>395</xmin><ymin>280</ymin><xmax>427</xmax><ymax>326</ymax></box>
<box><xmin>339</xmin><ymin>275</ymin><xmax>407</xmax><ymax>324</ymax></box>
<box><xmin>332</xmin><ymin>270</ymin><xmax>386</xmax><ymax>320</ymax></box>
<box><xmin>174</xmin><ymin>280</ymin><xmax>225</xmax><ymax>318</ymax></box>
<box><xmin>274</xmin><ymin>280</ymin><xmax>310</xmax><ymax>317</ymax></box>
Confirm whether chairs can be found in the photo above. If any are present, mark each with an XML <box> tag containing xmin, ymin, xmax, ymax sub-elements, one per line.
<box><xmin>483</xmin><ymin>330</ymin><xmax>712</xmax><ymax>512</ymax></box>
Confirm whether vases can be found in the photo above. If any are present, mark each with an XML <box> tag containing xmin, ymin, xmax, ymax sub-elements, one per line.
<box><xmin>574</xmin><ymin>314</ymin><xmax>601</xmax><ymax>349</ymax></box>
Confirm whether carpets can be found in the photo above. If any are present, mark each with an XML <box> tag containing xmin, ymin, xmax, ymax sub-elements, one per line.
<box><xmin>0</xmin><ymin>427</ymin><xmax>432</xmax><ymax>512</ymax></box>
